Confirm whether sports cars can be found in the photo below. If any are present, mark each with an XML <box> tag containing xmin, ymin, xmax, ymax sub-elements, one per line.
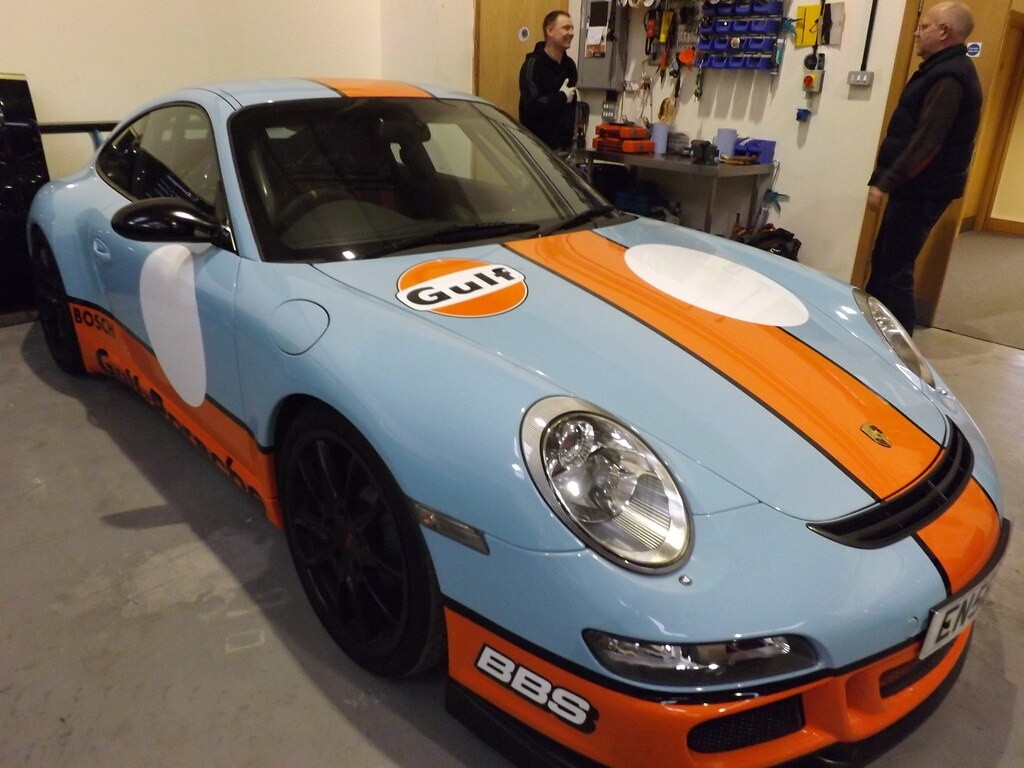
<box><xmin>25</xmin><ymin>75</ymin><xmax>1013</xmax><ymax>768</ymax></box>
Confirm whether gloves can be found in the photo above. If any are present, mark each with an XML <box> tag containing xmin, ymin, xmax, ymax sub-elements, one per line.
<box><xmin>560</xmin><ymin>78</ymin><xmax>577</xmax><ymax>104</ymax></box>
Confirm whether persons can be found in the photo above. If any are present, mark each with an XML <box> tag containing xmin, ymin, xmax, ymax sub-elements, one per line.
<box><xmin>519</xmin><ymin>10</ymin><xmax>578</xmax><ymax>162</ymax></box>
<box><xmin>864</xmin><ymin>1</ymin><xmax>983</xmax><ymax>337</ymax></box>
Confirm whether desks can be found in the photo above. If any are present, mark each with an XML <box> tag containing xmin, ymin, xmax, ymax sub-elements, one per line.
<box><xmin>574</xmin><ymin>150</ymin><xmax>775</xmax><ymax>233</ymax></box>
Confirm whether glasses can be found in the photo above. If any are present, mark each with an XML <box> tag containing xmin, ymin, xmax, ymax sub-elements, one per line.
<box><xmin>917</xmin><ymin>23</ymin><xmax>946</xmax><ymax>33</ymax></box>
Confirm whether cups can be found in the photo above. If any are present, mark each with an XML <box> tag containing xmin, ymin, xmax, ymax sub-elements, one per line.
<box><xmin>653</xmin><ymin>124</ymin><xmax>668</xmax><ymax>153</ymax></box>
<box><xmin>717</xmin><ymin>128</ymin><xmax>736</xmax><ymax>159</ymax></box>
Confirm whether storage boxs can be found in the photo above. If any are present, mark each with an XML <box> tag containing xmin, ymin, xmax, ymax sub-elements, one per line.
<box><xmin>616</xmin><ymin>192</ymin><xmax>664</xmax><ymax>219</ymax></box>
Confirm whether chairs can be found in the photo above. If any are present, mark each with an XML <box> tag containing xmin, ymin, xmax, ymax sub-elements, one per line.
<box><xmin>281</xmin><ymin>121</ymin><xmax>405</xmax><ymax>212</ymax></box>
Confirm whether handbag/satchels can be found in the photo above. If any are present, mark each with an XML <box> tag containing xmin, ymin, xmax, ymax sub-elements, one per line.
<box><xmin>749</xmin><ymin>227</ymin><xmax>802</xmax><ymax>261</ymax></box>
